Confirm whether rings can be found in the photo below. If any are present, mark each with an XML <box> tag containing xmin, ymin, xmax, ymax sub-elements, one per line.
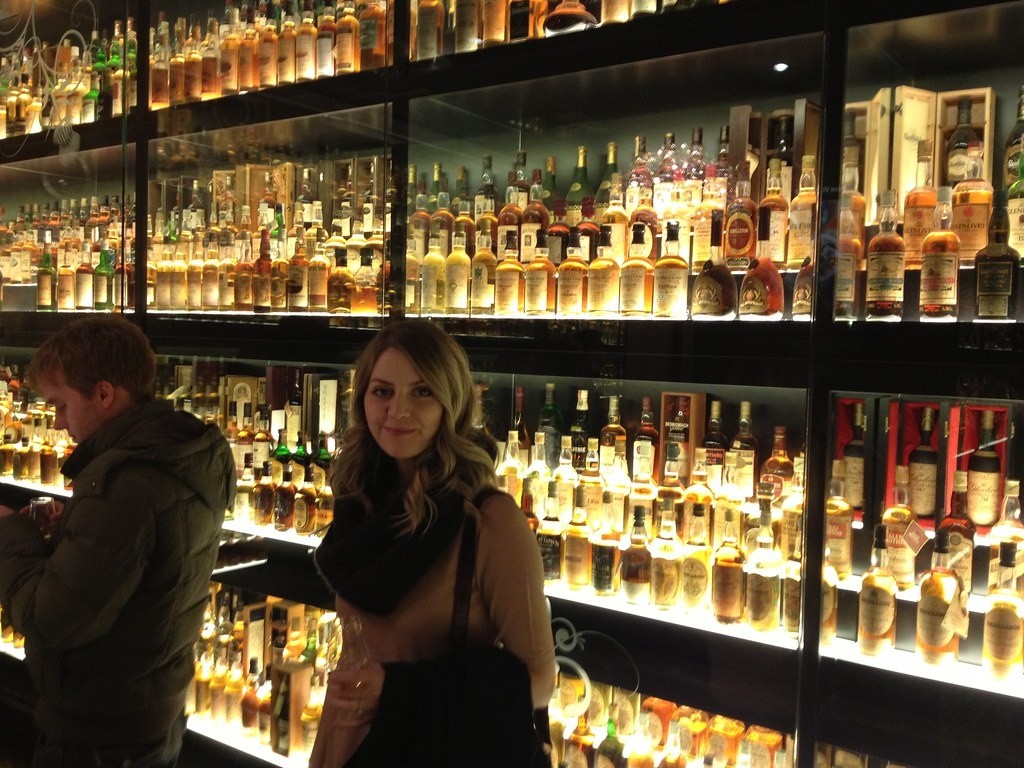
<box><xmin>357</xmin><ymin>707</ymin><xmax>364</xmax><ymax>719</ymax></box>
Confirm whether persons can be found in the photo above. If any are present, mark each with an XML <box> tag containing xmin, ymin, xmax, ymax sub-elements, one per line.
<box><xmin>0</xmin><ymin>314</ymin><xmax>237</xmax><ymax>768</ymax></box>
<box><xmin>309</xmin><ymin>320</ymin><xmax>554</xmax><ymax>767</ymax></box>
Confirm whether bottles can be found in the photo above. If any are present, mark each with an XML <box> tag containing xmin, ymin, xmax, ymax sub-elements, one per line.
<box><xmin>0</xmin><ymin>0</ymin><xmax>1023</xmax><ymax>321</ymax></box>
<box><xmin>0</xmin><ymin>351</ymin><xmax>1024</xmax><ymax>768</ymax></box>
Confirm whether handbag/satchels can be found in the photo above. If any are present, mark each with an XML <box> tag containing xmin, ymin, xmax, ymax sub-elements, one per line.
<box><xmin>347</xmin><ymin>485</ymin><xmax>552</xmax><ymax>768</ymax></box>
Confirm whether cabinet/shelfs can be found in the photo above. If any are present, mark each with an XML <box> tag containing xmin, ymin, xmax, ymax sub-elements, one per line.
<box><xmin>1</xmin><ymin>0</ymin><xmax>1024</xmax><ymax>767</ymax></box>
